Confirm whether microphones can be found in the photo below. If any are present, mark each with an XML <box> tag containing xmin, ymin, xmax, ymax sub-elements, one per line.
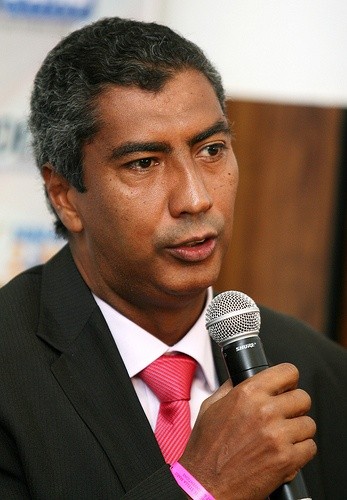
<box><xmin>206</xmin><ymin>290</ymin><xmax>312</xmax><ymax>500</ymax></box>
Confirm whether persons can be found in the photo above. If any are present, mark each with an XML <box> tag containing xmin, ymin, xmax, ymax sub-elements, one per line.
<box><xmin>168</xmin><ymin>460</ymin><xmax>217</xmax><ymax>500</ymax></box>
<box><xmin>0</xmin><ymin>15</ymin><xmax>347</xmax><ymax>500</ymax></box>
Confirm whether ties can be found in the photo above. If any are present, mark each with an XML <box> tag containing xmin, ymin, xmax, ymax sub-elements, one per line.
<box><xmin>137</xmin><ymin>351</ymin><xmax>200</xmax><ymax>467</ymax></box>
